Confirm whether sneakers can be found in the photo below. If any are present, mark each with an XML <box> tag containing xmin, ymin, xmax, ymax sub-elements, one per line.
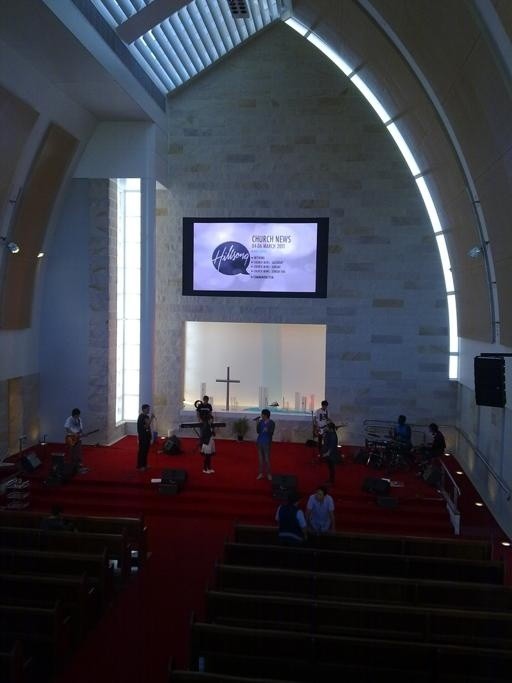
<box><xmin>267</xmin><ymin>474</ymin><xmax>272</xmax><ymax>481</ymax></box>
<box><xmin>257</xmin><ymin>473</ymin><xmax>263</xmax><ymax>480</ymax></box>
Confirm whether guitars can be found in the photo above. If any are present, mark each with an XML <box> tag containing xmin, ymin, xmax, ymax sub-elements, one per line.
<box><xmin>64</xmin><ymin>429</ymin><xmax>100</xmax><ymax>448</ymax></box>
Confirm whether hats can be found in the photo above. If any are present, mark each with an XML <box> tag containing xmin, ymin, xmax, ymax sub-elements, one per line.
<box><xmin>289</xmin><ymin>494</ymin><xmax>302</xmax><ymax>504</ymax></box>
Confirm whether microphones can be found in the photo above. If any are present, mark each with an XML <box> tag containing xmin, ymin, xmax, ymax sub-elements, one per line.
<box><xmin>253</xmin><ymin>416</ymin><xmax>260</xmax><ymax>420</ymax></box>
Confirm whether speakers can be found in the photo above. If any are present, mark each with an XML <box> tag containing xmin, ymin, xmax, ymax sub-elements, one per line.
<box><xmin>474</xmin><ymin>355</ymin><xmax>506</xmax><ymax>408</ymax></box>
<box><xmin>363</xmin><ymin>476</ymin><xmax>390</xmax><ymax>495</ymax></box>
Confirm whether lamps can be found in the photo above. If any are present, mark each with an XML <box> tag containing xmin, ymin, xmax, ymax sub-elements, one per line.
<box><xmin>0</xmin><ymin>235</ymin><xmax>21</xmax><ymax>254</ymax></box>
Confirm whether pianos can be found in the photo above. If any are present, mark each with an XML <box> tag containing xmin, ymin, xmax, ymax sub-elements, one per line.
<box><xmin>180</xmin><ymin>421</ymin><xmax>226</xmax><ymax>427</ymax></box>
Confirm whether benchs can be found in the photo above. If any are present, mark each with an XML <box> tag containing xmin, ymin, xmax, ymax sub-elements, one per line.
<box><xmin>0</xmin><ymin>504</ymin><xmax>150</xmax><ymax>682</ymax></box>
<box><xmin>167</xmin><ymin>517</ymin><xmax>510</xmax><ymax>682</ymax></box>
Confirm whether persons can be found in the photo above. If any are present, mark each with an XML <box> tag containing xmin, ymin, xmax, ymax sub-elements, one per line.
<box><xmin>415</xmin><ymin>422</ymin><xmax>446</xmax><ymax>477</ymax></box>
<box><xmin>255</xmin><ymin>408</ymin><xmax>275</xmax><ymax>481</ymax></box>
<box><xmin>135</xmin><ymin>403</ymin><xmax>155</xmax><ymax>471</ymax></box>
<box><xmin>196</xmin><ymin>395</ymin><xmax>213</xmax><ymax>421</ymax></box>
<box><xmin>200</xmin><ymin>415</ymin><xmax>216</xmax><ymax>474</ymax></box>
<box><xmin>325</xmin><ymin>423</ymin><xmax>340</xmax><ymax>485</ymax></box>
<box><xmin>315</xmin><ymin>400</ymin><xmax>329</xmax><ymax>457</ymax></box>
<box><xmin>275</xmin><ymin>489</ymin><xmax>308</xmax><ymax>547</ymax></box>
<box><xmin>64</xmin><ymin>408</ymin><xmax>89</xmax><ymax>474</ymax></box>
<box><xmin>393</xmin><ymin>414</ymin><xmax>411</xmax><ymax>451</ymax></box>
<box><xmin>305</xmin><ymin>488</ymin><xmax>338</xmax><ymax>532</ymax></box>
